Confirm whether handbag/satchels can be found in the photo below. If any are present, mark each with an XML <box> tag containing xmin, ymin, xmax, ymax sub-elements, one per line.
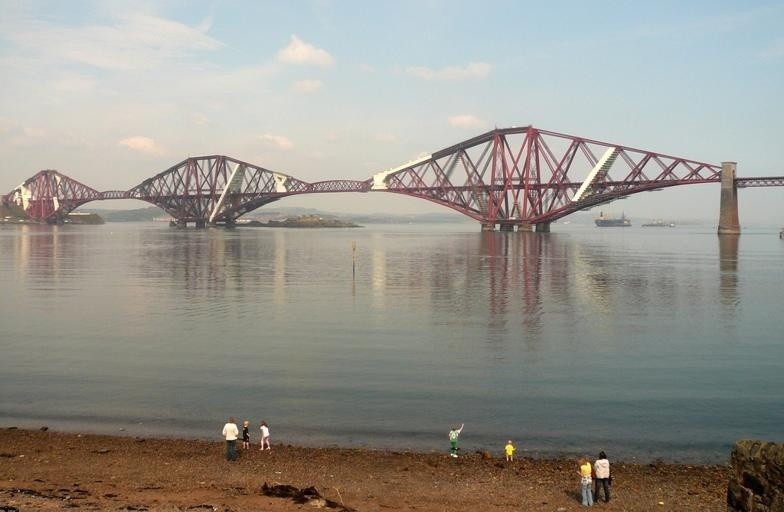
<box><xmin>591</xmin><ymin>468</ymin><xmax>597</xmax><ymax>479</ymax></box>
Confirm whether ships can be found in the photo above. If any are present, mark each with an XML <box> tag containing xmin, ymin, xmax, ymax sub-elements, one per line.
<box><xmin>641</xmin><ymin>218</ymin><xmax>676</xmax><ymax>228</ymax></box>
<box><xmin>595</xmin><ymin>211</ymin><xmax>632</xmax><ymax>227</ymax></box>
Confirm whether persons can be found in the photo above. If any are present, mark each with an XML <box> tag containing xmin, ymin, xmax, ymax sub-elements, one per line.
<box><xmin>448</xmin><ymin>422</ymin><xmax>465</xmax><ymax>457</ymax></box>
<box><xmin>593</xmin><ymin>450</ymin><xmax>610</xmax><ymax>502</ymax></box>
<box><xmin>241</xmin><ymin>420</ymin><xmax>251</xmax><ymax>449</ymax></box>
<box><xmin>221</xmin><ymin>417</ymin><xmax>239</xmax><ymax>461</ymax></box>
<box><xmin>576</xmin><ymin>456</ymin><xmax>594</xmax><ymax>507</ymax></box>
<box><xmin>503</xmin><ymin>439</ymin><xmax>517</xmax><ymax>462</ymax></box>
<box><xmin>258</xmin><ymin>419</ymin><xmax>271</xmax><ymax>451</ymax></box>
